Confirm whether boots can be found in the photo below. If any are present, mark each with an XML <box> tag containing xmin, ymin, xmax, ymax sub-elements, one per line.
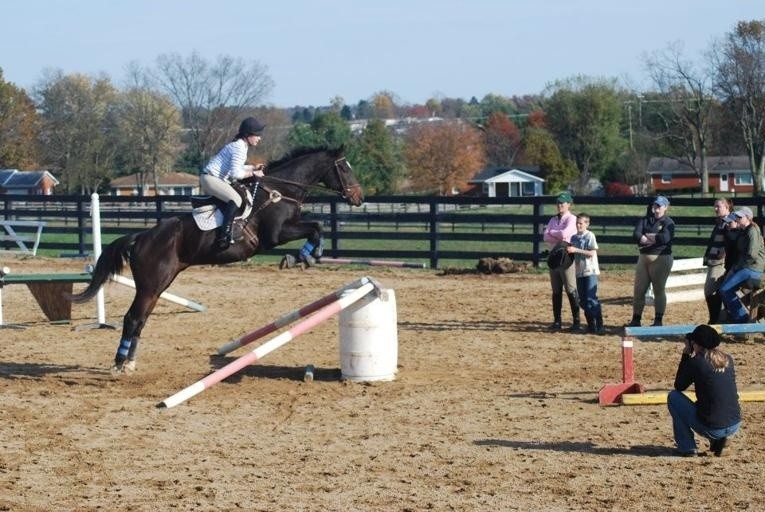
<box><xmin>548</xmin><ymin>289</ymin><xmax>662</xmax><ymax>335</ymax></box>
<box><xmin>218</xmin><ymin>200</ymin><xmax>240</xmax><ymax>248</ymax></box>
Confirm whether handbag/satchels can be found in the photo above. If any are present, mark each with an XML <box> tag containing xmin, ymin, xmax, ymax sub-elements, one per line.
<box><xmin>547</xmin><ymin>240</ymin><xmax>575</xmax><ymax>271</ymax></box>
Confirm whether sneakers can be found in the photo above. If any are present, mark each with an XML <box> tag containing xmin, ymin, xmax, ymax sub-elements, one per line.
<box><xmin>674</xmin><ymin>448</ymin><xmax>697</xmax><ymax>457</ymax></box>
<box><xmin>714</xmin><ymin>436</ymin><xmax>731</xmax><ymax>456</ymax></box>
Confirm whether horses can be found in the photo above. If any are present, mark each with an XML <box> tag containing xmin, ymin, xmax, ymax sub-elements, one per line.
<box><xmin>60</xmin><ymin>142</ymin><xmax>365</xmax><ymax>378</ymax></box>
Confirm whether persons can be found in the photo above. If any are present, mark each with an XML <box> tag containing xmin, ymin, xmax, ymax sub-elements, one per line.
<box><xmin>668</xmin><ymin>325</ymin><xmax>742</xmax><ymax>457</ymax></box>
<box><xmin>201</xmin><ymin>117</ymin><xmax>265</xmax><ymax>252</ymax></box>
<box><xmin>567</xmin><ymin>213</ymin><xmax>604</xmax><ymax>336</ymax></box>
<box><xmin>624</xmin><ymin>197</ymin><xmax>675</xmax><ymax>329</ymax></box>
<box><xmin>719</xmin><ymin>213</ymin><xmax>743</xmax><ymax>324</ymax></box>
<box><xmin>719</xmin><ymin>206</ymin><xmax>765</xmax><ymax>325</ymax></box>
<box><xmin>543</xmin><ymin>194</ymin><xmax>579</xmax><ymax>330</ymax></box>
<box><xmin>703</xmin><ymin>197</ymin><xmax>733</xmax><ymax>325</ymax></box>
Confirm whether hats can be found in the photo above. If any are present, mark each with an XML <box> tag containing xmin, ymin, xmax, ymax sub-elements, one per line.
<box><xmin>732</xmin><ymin>206</ymin><xmax>753</xmax><ymax>218</ymax></box>
<box><xmin>557</xmin><ymin>194</ymin><xmax>572</xmax><ymax>203</ymax></box>
<box><xmin>238</xmin><ymin>117</ymin><xmax>266</xmax><ymax>136</ymax></box>
<box><xmin>687</xmin><ymin>325</ymin><xmax>720</xmax><ymax>349</ymax></box>
<box><xmin>723</xmin><ymin>213</ymin><xmax>737</xmax><ymax>223</ymax></box>
<box><xmin>655</xmin><ymin>196</ymin><xmax>669</xmax><ymax>206</ymax></box>
